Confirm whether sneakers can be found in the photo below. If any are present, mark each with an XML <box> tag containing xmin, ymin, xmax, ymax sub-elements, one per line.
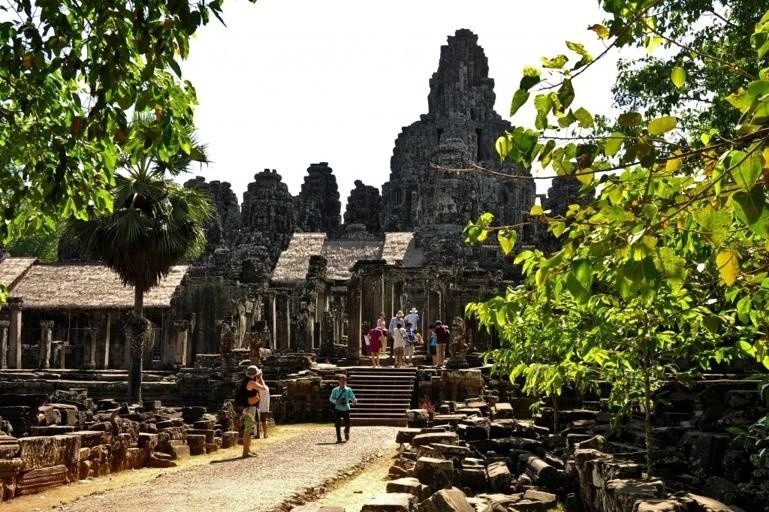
<box><xmin>344</xmin><ymin>429</ymin><xmax>349</xmax><ymax>440</ymax></box>
<box><xmin>253</xmin><ymin>435</ymin><xmax>268</xmax><ymax>439</ymax></box>
<box><xmin>243</xmin><ymin>451</ymin><xmax>258</xmax><ymax>457</ymax></box>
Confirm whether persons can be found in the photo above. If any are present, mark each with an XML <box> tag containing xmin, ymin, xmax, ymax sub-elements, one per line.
<box><xmin>329</xmin><ymin>374</ymin><xmax>357</xmax><ymax>443</ymax></box>
<box><xmin>236</xmin><ymin>365</ymin><xmax>269</xmax><ymax>458</ymax></box>
<box><xmin>367</xmin><ymin>307</ymin><xmax>450</xmax><ymax>369</ymax></box>
<box><xmin>253</xmin><ymin>385</ymin><xmax>270</xmax><ymax>438</ymax></box>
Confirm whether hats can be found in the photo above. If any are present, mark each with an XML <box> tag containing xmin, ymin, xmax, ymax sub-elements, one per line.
<box><xmin>396</xmin><ymin>310</ymin><xmax>404</xmax><ymax>317</ymax></box>
<box><xmin>410</xmin><ymin>307</ymin><xmax>418</xmax><ymax>312</ymax></box>
<box><xmin>245</xmin><ymin>365</ymin><xmax>262</xmax><ymax>378</ymax></box>
